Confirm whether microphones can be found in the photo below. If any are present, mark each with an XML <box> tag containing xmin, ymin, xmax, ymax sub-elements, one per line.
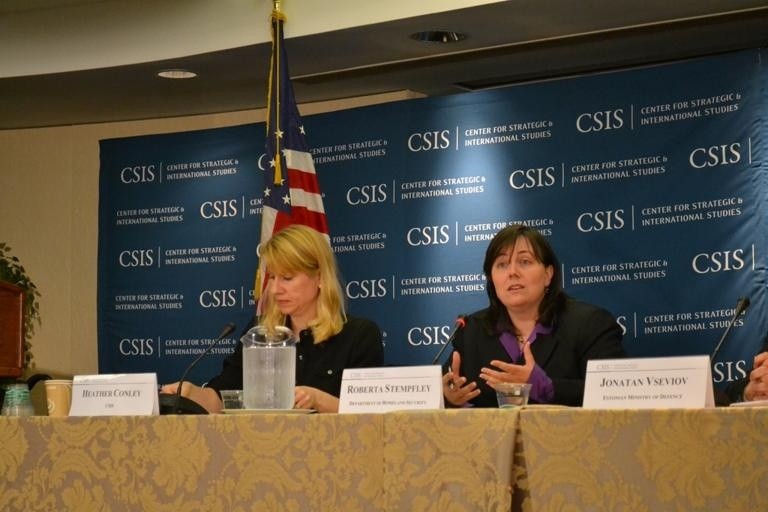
<box><xmin>156</xmin><ymin>320</ymin><xmax>238</xmax><ymax>412</ymax></box>
<box><xmin>432</xmin><ymin>310</ymin><xmax>469</xmax><ymax>367</ymax></box>
<box><xmin>709</xmin><ymin>294</ymin><xmax>751</xmax><ymax>406</ymax></box>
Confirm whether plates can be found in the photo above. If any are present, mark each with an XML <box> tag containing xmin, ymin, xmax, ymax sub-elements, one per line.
<box><xmin>729</xmin><ymin>398</ymin><xmax>768</xmax><ymax>407</ymax></box>
<box><xmin>217</xmin><ymin>408</ymin><xmax>317</xmax><ymax>415</ymax></box>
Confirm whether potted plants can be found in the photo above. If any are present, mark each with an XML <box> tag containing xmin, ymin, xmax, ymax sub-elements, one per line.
<box><xmin>0</xmin><ymin>238</ymin><xmax>42</xmax><ymax>409</ymax></box>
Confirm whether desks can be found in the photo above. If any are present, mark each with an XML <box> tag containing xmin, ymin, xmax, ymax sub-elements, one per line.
<box><xmin>518</xmin><ymin>403</ymin><xmax>768</xmax><ymax>512</ymax></box>
<box><xmin>0</xmin><ymin>407</ymin><xmax>521</xmax><ymax>512</ymax></box>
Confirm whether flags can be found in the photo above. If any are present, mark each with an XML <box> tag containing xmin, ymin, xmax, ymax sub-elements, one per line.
<box><xmin>250</xmin><ymin>13</ymin><xmax>340</xmax><ymax>319</ymax></box>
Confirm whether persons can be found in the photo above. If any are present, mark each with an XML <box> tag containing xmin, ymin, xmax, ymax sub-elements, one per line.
<box><xmin>440</xmin><ymin>226</ymin><xmax>626</xmax><ymax>407</ymax></box>
<box><xmin>158</xmin><ymin>227</ymin><xmax>382</xmax><ymax>413</ymax></box>
<box><xmin>741</xmin><ymin>350</ymin><xmax>768</xmax><ymax>407</ymax></box>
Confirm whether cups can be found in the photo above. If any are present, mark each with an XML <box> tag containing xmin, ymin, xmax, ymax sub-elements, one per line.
<box><xmin>241</xmin><ymin>327</ymin><xmax>297</xmax><ymax>410</ymax></box>
<box><xmin>496</xmin><ymin>383</ymin><xmax>531</xmax><ymax>410</ymax></box>
<box><xmin>220</xmin><ymin>389</ymin><xmax>244</xmax><ymax>409</ymax></box>
<box><xmin>45</xmin><ymin>379</ymin><xmax>73</xmax><ymax>417</ymax></box>
<box><xmin>2</xmin><ymin>383</ymin><xmax>34</xmax><ymax>415</ymax></box>
